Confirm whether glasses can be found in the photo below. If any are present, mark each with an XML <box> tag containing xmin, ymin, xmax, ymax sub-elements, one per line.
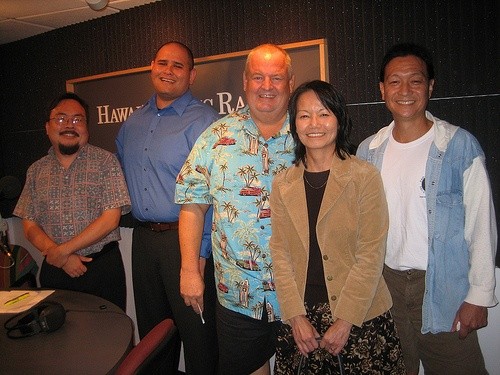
<box><xmin>47</xmin><ymin>115</ymin><xmax>87</xmax><ymax>126</ymax></box>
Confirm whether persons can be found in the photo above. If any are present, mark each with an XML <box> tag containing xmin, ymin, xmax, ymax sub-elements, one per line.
<box><xmin>174</xmin><ymin>43</ymin><xmax>297</xmax><ymax>375</ymax></box>
<box><xmin>112</xmin><ymin>41</ymin><xmax>221</xmax><ymax>375</ymax></box>
<box><xmin>355</xmin><ymin>43</ymin><xmax>500</xmax><ymax>375</ymax></box>
<box><xmin>12</xmin><ymin>92</ymin><xmax>131</xmax><ymax>314</ymax></box>
<box><xmin>270</xmin><ymin>80</ymin><xmax>406</xmax><ymax>375</ymax></box>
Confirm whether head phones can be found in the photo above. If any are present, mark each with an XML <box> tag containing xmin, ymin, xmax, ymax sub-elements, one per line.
<box><xmin>3</xmin><ymin>301</ymin><xmax>66</xmax><ymax>339</ymax></box>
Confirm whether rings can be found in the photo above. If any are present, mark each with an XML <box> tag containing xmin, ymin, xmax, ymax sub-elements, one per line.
<box><xmin>330</xmin><ymin>347</ymin><xmax>335</xmax><ymax>351</ymax></box>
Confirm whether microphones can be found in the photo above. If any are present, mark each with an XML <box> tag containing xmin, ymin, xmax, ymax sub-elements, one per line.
<box><xmin>0</xmin><ymin>219</ymin><xmax>12</xmax><ymax>258</ymax></box>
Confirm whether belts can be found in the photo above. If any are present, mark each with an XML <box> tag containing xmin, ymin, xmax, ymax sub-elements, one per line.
<box><xmin>151</xmin><ymin>223</ymin><xmax>177</xmax><ymax>232</ymax></box>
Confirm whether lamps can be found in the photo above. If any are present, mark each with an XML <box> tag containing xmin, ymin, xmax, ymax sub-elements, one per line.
<box><xmin>85</xmin><ymin>0</ymin><xmax>108</xmax><ymax>11</ymax></box>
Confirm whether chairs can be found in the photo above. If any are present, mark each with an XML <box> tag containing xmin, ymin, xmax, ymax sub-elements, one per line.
<box><xmin>115</xmin><ymin>319</ymin><xmax>182</xmax><ymax>375</ymax></box>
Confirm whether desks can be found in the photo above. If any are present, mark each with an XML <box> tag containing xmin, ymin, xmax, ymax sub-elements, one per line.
<box><xmin>0</xmin><ymin>290</ymin><xmax>134</xmax><ymax>375</ymax></box>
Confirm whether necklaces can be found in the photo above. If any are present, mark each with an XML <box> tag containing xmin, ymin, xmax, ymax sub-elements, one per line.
<box><xmin>305</xmin><ymin>176</ymin><xmax>328</xmax><ymax>189</ymax></box>
